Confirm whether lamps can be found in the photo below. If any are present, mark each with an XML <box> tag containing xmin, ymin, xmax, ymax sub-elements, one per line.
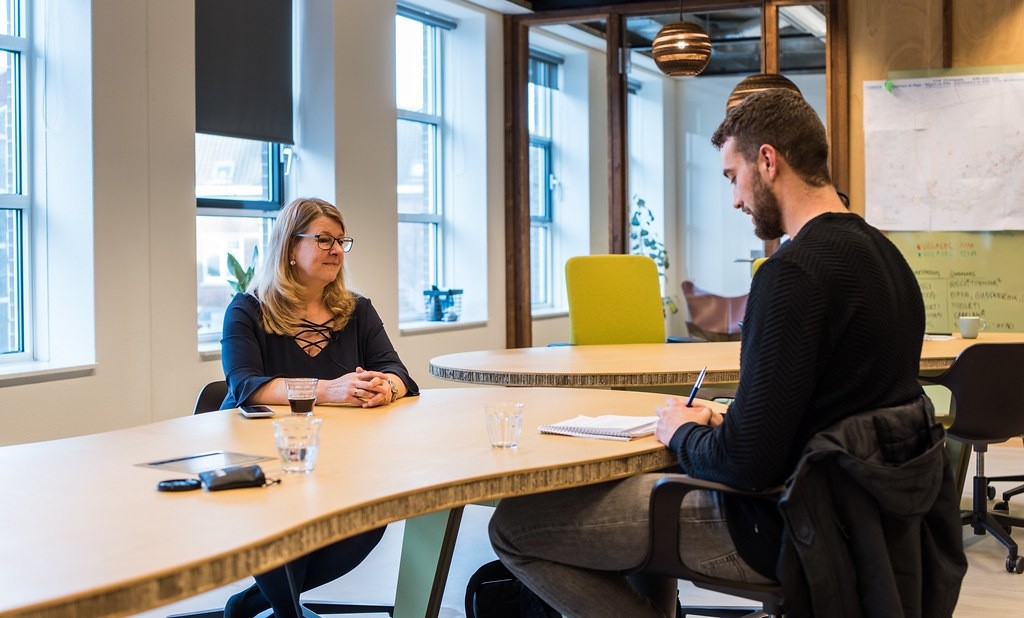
<box><xmin>653</xmin><ymin>1</ymin><xmax>714</xmax><ymax>78</ymax></box>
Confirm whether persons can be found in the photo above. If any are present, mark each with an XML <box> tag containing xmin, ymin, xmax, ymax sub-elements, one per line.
<box><xmin>224</xmin><ymin>197</ymin><xmax>420</xmax><ymax>618</ymax></box>
<box><xmin>488</xmin><ymin>91</ymin><xmax>925</xmax><ymax>618</ymax></box>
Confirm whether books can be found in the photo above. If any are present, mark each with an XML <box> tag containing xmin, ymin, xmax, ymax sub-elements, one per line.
<box><xmin>538</xmin><ymin>413</ymin><xmax>660</xmax><ymax>442</ymax></box>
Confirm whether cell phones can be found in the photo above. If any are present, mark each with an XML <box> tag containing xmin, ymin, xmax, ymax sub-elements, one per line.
<box><xmin>239</xmin><ymin>405</ymin><xmax>276</xmax><ymax>417</ymax></box>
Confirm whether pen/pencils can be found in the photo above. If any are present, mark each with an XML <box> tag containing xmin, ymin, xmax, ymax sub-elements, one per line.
<box><xmin>686</xmin><ymin>366</ymin><xmax>707</xmax><ymax>407</ymax></box>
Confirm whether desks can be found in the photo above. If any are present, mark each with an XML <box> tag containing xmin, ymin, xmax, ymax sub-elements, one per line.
<box><xmin>0</xmin><ymin>384</ymin><xmax>728</xmax><ymax>618</ymax></box>
<box><xmin>428</xmin><ymin>331</ymin><xmax>1024</xmax><ymax>506</ymax></box>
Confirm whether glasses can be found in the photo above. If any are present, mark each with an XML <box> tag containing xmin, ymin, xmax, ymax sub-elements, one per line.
<box><xmin>296</xmin><ymin>234</ymin><xmax>354</xmax><ymax>253</ymax></box>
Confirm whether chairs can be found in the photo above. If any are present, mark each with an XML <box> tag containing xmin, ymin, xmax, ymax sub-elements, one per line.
<box><xmin>564</xmin><ymin>254</ymin><xmax>666</xmax><ymax>345</ymax></box>
<box><xmin>680</xmin><ymin>280</ymin><xmax>750</xmax><ymax>342</ymax></box>
<box><xmin>918</xmin><ymin>342</ymin><xmax>1024</xmax><ymax>573</ymax></box>
<box><xmin>621</xmin><ymin>475</ymin><xmax>786</xmax><ymax>618</ymax></box>
<box><xmin>164</xmin><ymin>380</ymin><xmax>395</xmax><ymax>618</ymax></box>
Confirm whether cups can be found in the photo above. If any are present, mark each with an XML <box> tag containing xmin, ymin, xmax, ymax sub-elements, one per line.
<box><xmin>959</xmin><ymin>317</ymin><xmax>986</xmax><ymax>339</ymax></box>
<box><xmin>284</xmin><ymin>378</ymin><xmax>318</xmax><ymax>416</ymax></box>
<box><xmin>485</xmin><ymin>402</ymin><xmax>525</xmax><ymax>449</ymax></box>
<box><xmin>272</xmin><ymin>416</ymin><xmax>323</xmax><ymax>474</ymax></box>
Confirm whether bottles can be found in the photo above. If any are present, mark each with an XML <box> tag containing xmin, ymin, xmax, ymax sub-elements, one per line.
<box><xmin>428</xmin><ymin>286</ymin><xmax>453</xmax><ymax>322</ymax></box>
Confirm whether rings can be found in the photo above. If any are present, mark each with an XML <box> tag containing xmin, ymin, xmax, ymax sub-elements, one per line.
<box><xmin>352</xmin><ymin>388</ymin><xmax>358</xmax><ymax>395</ymax></box>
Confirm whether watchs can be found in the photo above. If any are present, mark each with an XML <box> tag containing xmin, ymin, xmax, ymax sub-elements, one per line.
<box><xmin>387</xmin><ymin>380</ymin><xmax>398</xmax><ymax>401</ymax></box>
